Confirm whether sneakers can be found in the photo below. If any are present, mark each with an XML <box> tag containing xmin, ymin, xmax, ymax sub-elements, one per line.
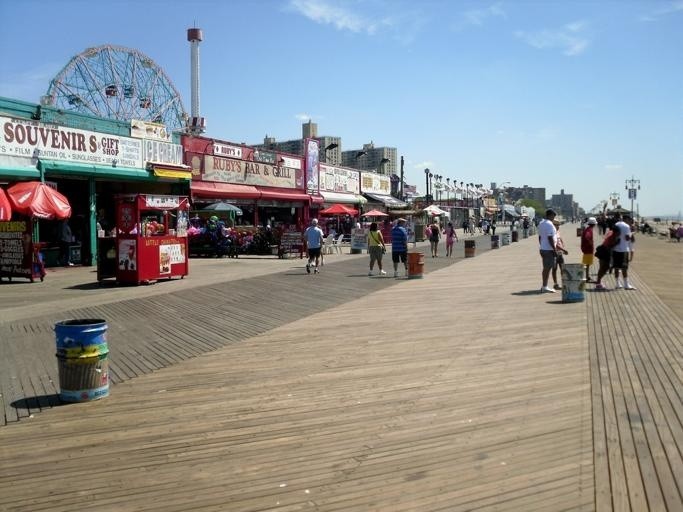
<box><xmin>554</xmin><ymin>285</ymin><xmax>561</xmax><ymax>289</ymax></box>
<box><xmin>369</xmin><ymin>269</ymin><xmax>408</xmax><ymax>277</ymax></box>
<box><xmin>585</xmin><ymin>277</ymin><xmax>637</xmax><ymax>290</ymax></box>
<box><xmin>541</xmin><ymin>287</ymin><xmax>555</xmax><ymax>292</ymax></box>
<box><xmin>306</xmin><ymin>262</ymin><xmax>323</xmax><ymax>274</ymax></box>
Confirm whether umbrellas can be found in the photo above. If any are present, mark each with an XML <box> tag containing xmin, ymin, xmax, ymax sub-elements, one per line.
<box><xmin>319</xmin><ymin>204</ymin><xmax>359</xmax><ymax>230</ymax></box>
<box><xmin>7</xmin><ymin>180</ymin><xmax>73</xmax><ymax>222</ymax></box>
<box><xmin>204</xmin><ymin>202</ymin><xmax>243</xmax><ymax>216</ymax></box>
<box><xmin>0</xmin><ymin>185</ymin><xmax>12</xmax><ymax>222</ymax></box>
<box><xmin>423</xmin><ymin>204</ymin><xmax>445</xmax><ymax>216</ymax></box>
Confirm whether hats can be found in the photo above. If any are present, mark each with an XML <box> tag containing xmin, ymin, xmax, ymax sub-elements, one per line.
<box><xmin>587</xmin><ymin>217</ymin><xmax>598</xmax><ymax>224</ymax></box>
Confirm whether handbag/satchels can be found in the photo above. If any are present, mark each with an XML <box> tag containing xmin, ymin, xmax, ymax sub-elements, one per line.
<box><xmin>424</xmin><ymin>227</ymin><xmax>432</xmax><ymax>237</ymax></box>
<box><xmin>382</xmin><ymin>246</ymin><xmax>386</xmax><ymax>255</ymax></box>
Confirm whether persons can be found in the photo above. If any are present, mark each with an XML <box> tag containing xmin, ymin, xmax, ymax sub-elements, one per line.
<box><xmin>425</xmin><ymin>221</ymin><xmax>458</xmax><ymax>258</ymax></box>
<box><xmin>640</xmin><ymin>220</ymin><xmax>683</xmax><ymax>241</ymax></box>
<box><xmin>463</xmin><ymin>214</ymin><xmax>496</xmax><ymax>236</ymax></box>
<box><xmin>390</xmin><ymin>217</ymin><xmax>409</xmax><ymax>277</ymax></box>
<box><xmin>513</xmin><ymin>215</ymin><xmax>543</xmax><ymax>238</ymax></box>
<box><xmin>594</xmin><ymin>214</ymin><xmax>635</xmax><ymax>291</ymax></box>
<box><xmin>305</xmin><ymin>218</ymin><xmax>325</xmax><ymax>275</ymax></box>
<box><xmin>579</xmin><ymin>216</ymin><xmax>598</xmax><ymax>283</ymax></box>
<box><xmin>55</xmin><ymin>221</ymin><xmax>76</xmax><ymax>267</ymax></box>
<box><xmin>538</xmin><ymin>210</ymin><xmax>568</xmax><ymax>292</ymax></box>
<box><xmin>190</xmin><ymin>212</ymin><xmax>201</xmax><ymax>220</ymax></box>
<box><xmin>367</xmin><ymin>223</ymin><xmax>388</xmax><ymax>275</ymax></box>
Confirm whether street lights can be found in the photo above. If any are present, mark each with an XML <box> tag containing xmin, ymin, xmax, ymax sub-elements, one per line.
<box><xmin>424</xmin><ymin>168</ymin><xmax>483</xmax><ymax>230</ymax></box>
<box><xmin>608</xmin><ymin>191</ymin><xmax>619</xmax><ymax>209</ymax></box>
<box><xmin>355</xmin><ymin>153</ymin><xmax>366</xmax><ymax>171</ymax></box>
<box><xmin>323</xmin><ymin>144</ymin><xmax>337</xmax><ymax>163</ymax></box>
<box><xmin>496</xmin><ymin>181</ymin><xmax>511</xmax><ymax>223</ymax></box>
<box><xmin>625</xmin><ymin>175</ymin><xmax>640</xmax><ymax>221</ymax></box>
<box><xmin>378</xmin><ymin>158</ymin><xmax>389</xmax><ymax>175</ymax></box>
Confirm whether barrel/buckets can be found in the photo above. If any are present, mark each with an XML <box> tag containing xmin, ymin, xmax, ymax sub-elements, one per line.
<box><xmin>522</xmin><ymin>229</ymin><xmax>529</xmax><ymax>238</ymax></box>
<box><xmin>502</xmin><ymin>232</ymin><xmax>512</xmax><ymax>246</ymax></box>
<box><xmin>464</xmin><ymin>239</ymin><xmax>476</xmax><ymax>258</ymax></box>
<box><xmin>562</xmin><ymin>262</ymin><xmax>586</xmax><ymax>303</ymax></box>
<box><xmin>512</xmin><ymin>231</ymin><xmax>518</xmax><ymax>242</ymax></box>
<box><xmin>490</xmin><ymin>235</ymin><xmax>500</xmax><ymax>249</ymax></box>
<box><xmin>405</xmin><ymin>252</ymin><xmax>425</xmax><ymax>279</ymax></box>
<box><xmin>53</xmin><ymin>318</ymin><xmax>111</xmax><ymax>402</ymax></box>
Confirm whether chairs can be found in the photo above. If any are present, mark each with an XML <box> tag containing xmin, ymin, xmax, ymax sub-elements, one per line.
<box><xmin>320</xmin><ymin>233</ymin><xmax>344</xmax><ymax>255</ymax></box>
